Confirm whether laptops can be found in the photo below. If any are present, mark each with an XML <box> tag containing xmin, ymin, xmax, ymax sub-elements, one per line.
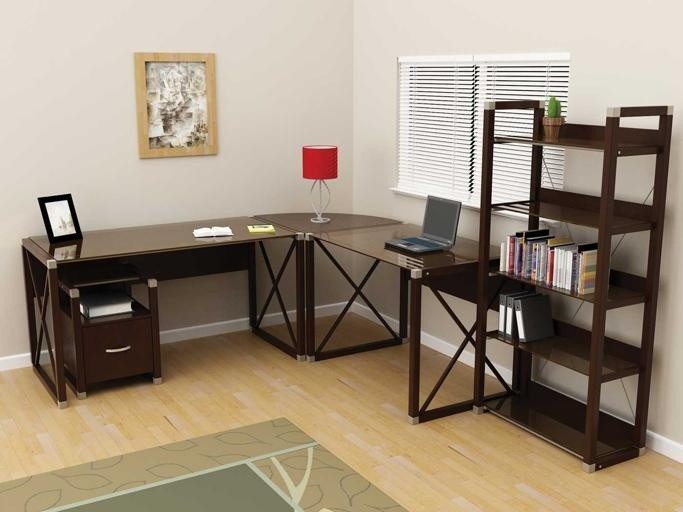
<box><xmin>384</xmin><ymin>195</ymin><xmax>461</xmax><ymax>254</ymax></box>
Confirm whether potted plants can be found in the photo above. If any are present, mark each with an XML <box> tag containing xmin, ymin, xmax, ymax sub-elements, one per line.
<box><xmin>542</xmin><ymin>97</ymin><xmax>563</xmax><ymax>143</ymax></box>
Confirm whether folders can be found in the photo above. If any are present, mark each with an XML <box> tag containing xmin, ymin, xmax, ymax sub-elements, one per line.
<box><xmin>506</xmin><ymin>294</ymin><xmax>543</xmax><ymax>339</ymax></box>
<box><xmin>515</xmin><ymin>295</ymin><xmax>554</xmax><ymax>342</ymax></box>
<box><xmin>498</xmin><ymin>291</ymin><xmax>532</xmax><ymax>336</ymax></box>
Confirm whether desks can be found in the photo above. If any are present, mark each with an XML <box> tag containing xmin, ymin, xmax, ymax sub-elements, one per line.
<box><xmin>22</xmin><ymin>212</ymin><xmax>524</xmax><ymax>423</ymax></box>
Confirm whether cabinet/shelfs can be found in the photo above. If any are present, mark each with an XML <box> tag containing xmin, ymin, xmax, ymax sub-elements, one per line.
<box><xmin>473</xmin><ymin>100</ymin><xmax>674</xmax><ymax>474</ymax></box>
<box><xmin>60</xmin><ymin>268</ymin><xmax>163</xmax><ymax>400</ymax></box>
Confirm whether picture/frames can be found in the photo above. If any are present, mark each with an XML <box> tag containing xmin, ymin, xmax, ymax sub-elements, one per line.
<box><xmin>48</xmin><ymin>245</ymin><xmax>82</xmax><ymax>260</ymax></box>
<box><xmin>135</xmin><ymin>52</ymin><xmax>218</xmax><ymax>161</ymax></box>
<box><xmin>37</xmin><ymin>194</ymin><xmax>84</xmax><ymax>243</ymax></box>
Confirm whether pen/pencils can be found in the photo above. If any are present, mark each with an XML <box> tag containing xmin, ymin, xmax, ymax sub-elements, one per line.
<box><xmin>253</xmin><ymin>226</ymin><xmax>270</xmax><ymax>228</ymax></box>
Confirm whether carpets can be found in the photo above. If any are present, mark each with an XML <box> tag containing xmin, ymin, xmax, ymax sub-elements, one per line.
<box><xmin>0</xmin><ymin>416</ymin><xmax>410</xmax><ymax>512</ymax></box>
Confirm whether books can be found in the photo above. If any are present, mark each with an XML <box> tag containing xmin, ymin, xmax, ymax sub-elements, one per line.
<box><xmin>80</xmin><ymin>291</ymin><xmax>136</xmax><ymax>320</ymax></box>
<box><xmin>194</xmin><ymin>227</ymin><xmax>235</xmax><ymax>237</ymax></box>
<box><xmin>499</xmin><ymin>229</ymin><xmax>610</xmax><ymax>295</ymax></box>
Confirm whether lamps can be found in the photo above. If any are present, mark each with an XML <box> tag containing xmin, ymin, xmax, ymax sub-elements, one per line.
<box><xmin>302</xmin><ymin>145</ymin><xmax>337</xmax><ymax>223</ymax></box>
